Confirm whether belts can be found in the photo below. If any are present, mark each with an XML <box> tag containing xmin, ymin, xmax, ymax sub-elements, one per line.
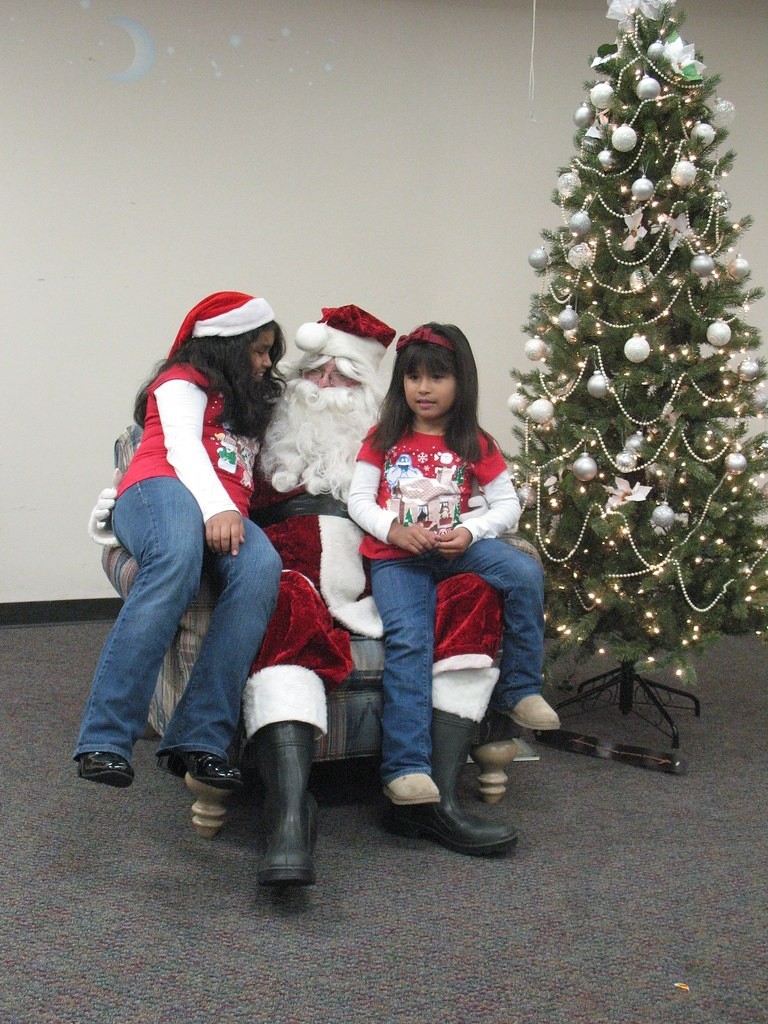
<box><xmin>252</xmin><ymin>489</ymin><xmax>352</xmax><ymax>527</ymax></box>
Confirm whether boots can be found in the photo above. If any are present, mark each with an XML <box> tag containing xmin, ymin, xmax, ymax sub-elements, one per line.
<box><xmin>380</xmin><ymin>663</ymin><xmax>519</xmax><ymax>856</ymax></box>
<box><xmin>241</xmin><ymin>664</ymin><xmax>320</xmax><ymax>886</ymax></box>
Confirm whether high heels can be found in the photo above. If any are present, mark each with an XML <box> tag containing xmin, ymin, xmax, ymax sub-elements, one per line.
<box><xmin>77</xmin><ymin>751</ymin><xmax>134</xmax><ymax>787</ymax></box>
<box><xmin>157</xmin><ymin>750</ymin><xmax>244</xmax><ymax>790</ymax></box>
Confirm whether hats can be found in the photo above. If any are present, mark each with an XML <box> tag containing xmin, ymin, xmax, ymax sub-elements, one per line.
<box><xmin>166</xmin><ymin>292</ymin><xmax>274</xmax><ymax>361</ymax></box>
<box><xmin>293</xmin><ymin>303</ymin><xmax>397</xmax><ymax>373</ymax></box>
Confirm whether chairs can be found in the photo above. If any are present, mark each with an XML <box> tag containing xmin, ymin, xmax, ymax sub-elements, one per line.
<box><xmin>100</xmin><ymin>427</ymin><xmax>542</xmax><ymax>839</ymax></box>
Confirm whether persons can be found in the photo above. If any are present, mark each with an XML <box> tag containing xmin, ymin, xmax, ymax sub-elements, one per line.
<box><xmin>87</xmin><ymin>305</ymin><xmax>517</xmax><ymax>887</ymax></box>
<box><xmin>347</xmin><ymin>323</ymin><xmax>561</xmax><ymax>805</ymax></box>
<box><xmin>73</xmin><ymin>291</ymin><xmax>286</xmax><ymax>789</ymax></box>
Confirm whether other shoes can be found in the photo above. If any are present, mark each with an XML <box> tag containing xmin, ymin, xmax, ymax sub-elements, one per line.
<box><xmin>384</xmin><ymin>774</ymin><xmax>441</xmax><ymax>806</ymax></box>
<box><xmin>494</xmin><ymin>695</ymin><xmax>561</xmax><ymax>730</ymax></box>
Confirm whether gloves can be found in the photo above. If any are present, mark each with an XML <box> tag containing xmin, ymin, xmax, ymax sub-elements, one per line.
<box><xmin>459</xmin><ymin>495</ymin><xmax>488</xmax><ymax>522</ymax></box>
<box><xmin>92</xmin><ymin>469</ymin><xmax>123</xmax><ymax>530</ymax></box>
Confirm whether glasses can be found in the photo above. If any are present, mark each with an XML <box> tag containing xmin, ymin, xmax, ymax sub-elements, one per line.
<box><xmin>299</xmin><ymin>367</ymin><xmax>354</xmax><ymax>387</ymax></box>
